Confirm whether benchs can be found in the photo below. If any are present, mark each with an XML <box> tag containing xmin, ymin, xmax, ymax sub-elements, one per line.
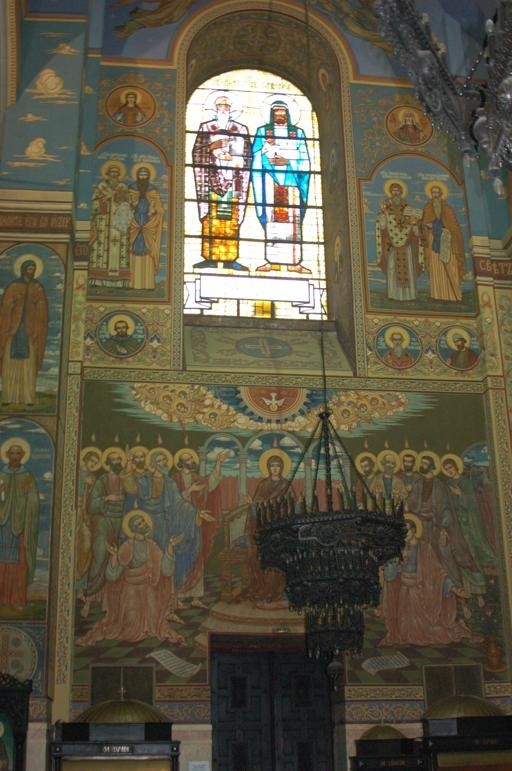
<box><xmin>347</xmin><ymin>716</ymin><xmax>510</xmax><ymax>771</ymax></box>
<box><xmin>46</xmin><ymin>721</ymin><xmax>180</xmax><ymax>771</ymax></box>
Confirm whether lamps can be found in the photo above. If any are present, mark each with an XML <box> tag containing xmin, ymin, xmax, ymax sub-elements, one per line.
<box><xmin>250</xmin><ymin>0</ymin><xmax>407</xmax><ymax>660</ymax></box>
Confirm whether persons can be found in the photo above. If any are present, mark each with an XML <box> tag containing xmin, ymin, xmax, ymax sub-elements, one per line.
<box><xmin>374</xmin><ymin>179</ymin><xmax>424</xmax><ymax>301</ymax></box>
<box><xmin>103</xmin><ymin>314</ymin><xmax>139</xmax><ymax>353</ymax></box>
<box><xmin>421</xmin><ymin>181</ymin><xmax>472</xmax><ymax>303</ymax></box>
<box><xmin>90</xmin><ymin>164</ymin><xmax>133</xmax><ymax>288</ymax></box>
<box><xmin>379</xmin><ymin>326</ymin><xmax>416</xmax><ymax>367</ymax></box>
<box><xmin>125</xmin><ymin>163</ymin><xmax>163</xmax><ymax>291</ymax></box>
<box><xmin>442</xmin><ymin>328</ymin><xmax>477</xmax><ymax>369</ymax></box>
<box><xmin>251</xmin><ymin>100</ymin><xmax>311</xmax><ymax>274</ymax></box>
<box><xmin>1</xmin><ymin>260</ymin><xmax>48</xmax><ymax>404</ymax></box>
<box><xmin>113</xmin><ymin>91</ymin><xmax>145</xmax><ymax>124</ymax></box>
<box><xmin>1</xmin><ymin>446</ymin><xmax>42</xmax><ymax>613</ymax></box>
<box><xmin>191</xmin><ymin>95</ymin><xmax>249</xmax><ymax>270</ymax></box>
<box><xmin>396</xmin><ymin>110</ymin><xmax>426</xmax><ymax>144</ymax></box>
